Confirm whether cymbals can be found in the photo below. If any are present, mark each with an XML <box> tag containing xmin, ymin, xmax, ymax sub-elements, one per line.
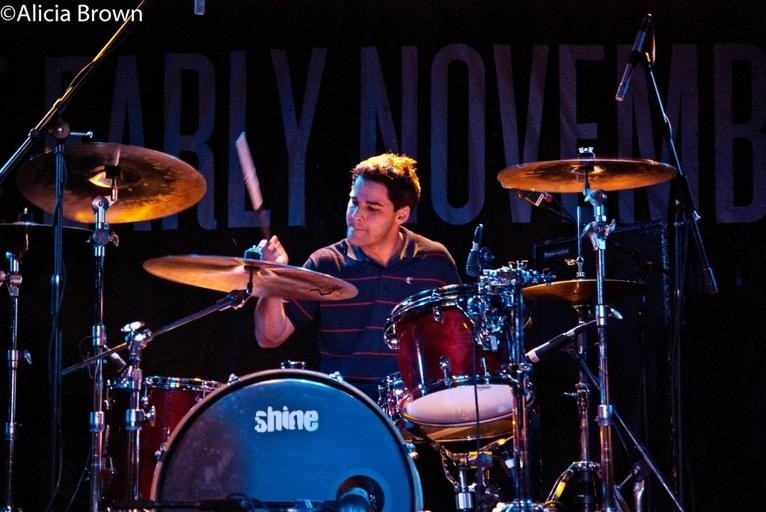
<box><xmin>496</xmin><ymin>158</ymin><xmax>677</xmax><ymax>194</ymax></box>
<box><xmin>17</xmin><ymin>143</ymin><xmax>206</xmax><ymax>224</ymax></box>
<box><xmin>520</xmin><ymin>280</ymin><xmax>645</xmax><ymax>305</ymax></box>
<box><xmin>146</xmin><ymin>255</ymin><xmax>359</xmax><ymax>303</ymax></box>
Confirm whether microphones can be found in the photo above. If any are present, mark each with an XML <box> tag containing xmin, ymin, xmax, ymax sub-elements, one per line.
<box><xmin>462</xmin><ymin>224</ymin><xmax>486</xmax><ymax>276</ymax></box>
<box><xmin>612</xmin><ymin>1</ymin><xmax>669</xmax><ymax>103</ymax></box>
<box><xmin>188</xmin><ymin>0</ymin><xmax>208</xmax><ymax>17</ymax></box>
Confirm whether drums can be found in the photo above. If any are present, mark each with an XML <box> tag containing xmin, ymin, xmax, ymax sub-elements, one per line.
<box><xmin>135</xmin><ymin>377</ymin><xmax>231</xmax><ymax>508</ymax></box>
<box><xmin>383</xmin><ymin>282</ymin><xmax>536</xmax><ymax>429</ymax></box>
<box><xmin>150</xmin><ymin>362</ymin><xmax>423</xmax><ymax>512</ymax></box>
<box><xmin>379</xmin><ymin>367</ymin><xmax>542</xmax><ymax>443</ymax></box>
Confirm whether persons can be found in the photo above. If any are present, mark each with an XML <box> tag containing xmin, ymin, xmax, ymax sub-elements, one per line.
<box><xmin>255</xmin><ymin>154</ymin><xmax>468</xmax><ymax>415</ymax></box>
